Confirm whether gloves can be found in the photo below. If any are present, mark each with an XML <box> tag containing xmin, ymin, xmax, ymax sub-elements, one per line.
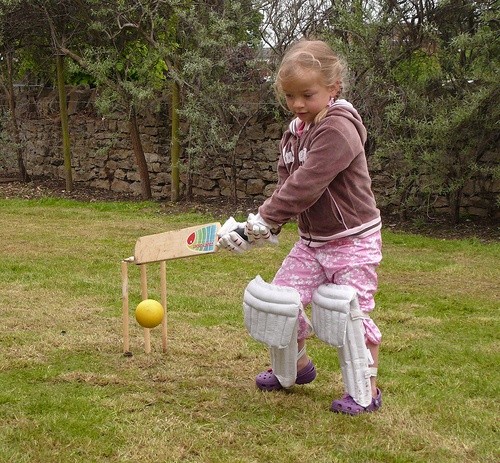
<box><xmin>214</xmin><ymin>216</ymin><xmax>255</xmax><ymax>253</ymax></box>
<box><xmin>239</xmin><ymin>213</ymin><xmax>279</xmax><ymax>249</ymax></box>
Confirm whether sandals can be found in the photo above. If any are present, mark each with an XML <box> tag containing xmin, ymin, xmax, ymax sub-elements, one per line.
<box><xmin>331</xmin><ymin>386</ymin><xmax>382</xmax><ymax>416</ymax></box>
<box><xmin>255</xmin><ymin>357</ymin><xmax>316</xmax><ymax>392</ymax></box>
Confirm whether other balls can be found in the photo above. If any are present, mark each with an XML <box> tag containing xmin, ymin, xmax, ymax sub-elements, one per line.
<box><xmin>134</xmin><ymin>298</ymin><xmax>165</xmax><ymax>330</ymax></box>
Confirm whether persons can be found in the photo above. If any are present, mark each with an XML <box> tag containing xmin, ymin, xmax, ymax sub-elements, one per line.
<box><xmin>215</xmin><ymin>40</ymin><xmax>384</xmax><ymax>415</ymax></box>
